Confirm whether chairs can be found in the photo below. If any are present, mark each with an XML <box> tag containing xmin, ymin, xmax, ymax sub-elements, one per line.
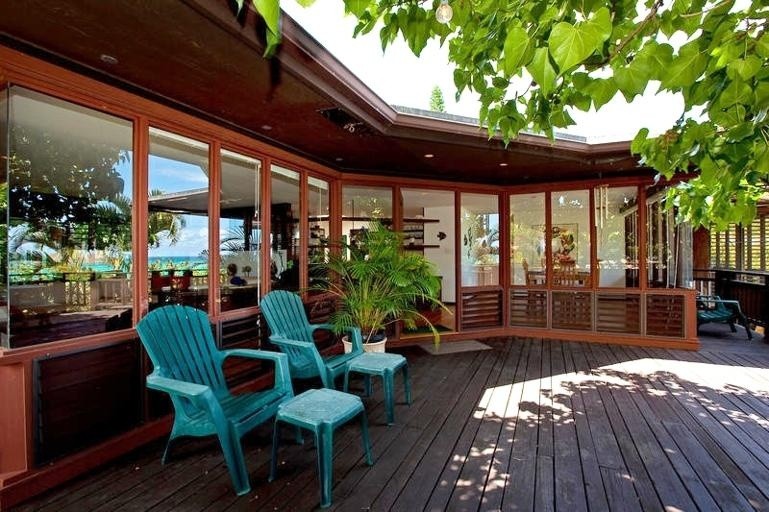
<box><xmin>522</xmin><ymin>260</ymin><xmax>602</xmax><ymax>322</ymax></box>
<box><xmin>672</xmin><ymin>278</ymin><xmax>754</xmax><ymax>341</ymax></box>
<box><xmin>260</xmin><ymin>289</ymin><xmax>371</xmax><ymax>444</ymax></box>
<box><xmin>136</xmin><ymin>304</ymin><xmax>303</xmax><ymax>495</ymax></box>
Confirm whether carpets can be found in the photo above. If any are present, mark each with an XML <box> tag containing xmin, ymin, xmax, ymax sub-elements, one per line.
<box><xmin>417</xmin><ymin>339</ymin><xmax>495</xmax><ymax>356</ymax></box>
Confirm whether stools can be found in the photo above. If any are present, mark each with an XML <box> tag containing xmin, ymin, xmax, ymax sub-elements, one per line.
<box><xmin>268</xmin><ymin>353</ymin><xmax>412</xmax><ymax>508</ymax></box>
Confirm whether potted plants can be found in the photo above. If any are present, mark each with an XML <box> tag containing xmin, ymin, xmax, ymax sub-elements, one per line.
<box><xmin>298</xmin><ymin>229</ymin><xmax>443</xmax><ymax>353</ymax></box>
